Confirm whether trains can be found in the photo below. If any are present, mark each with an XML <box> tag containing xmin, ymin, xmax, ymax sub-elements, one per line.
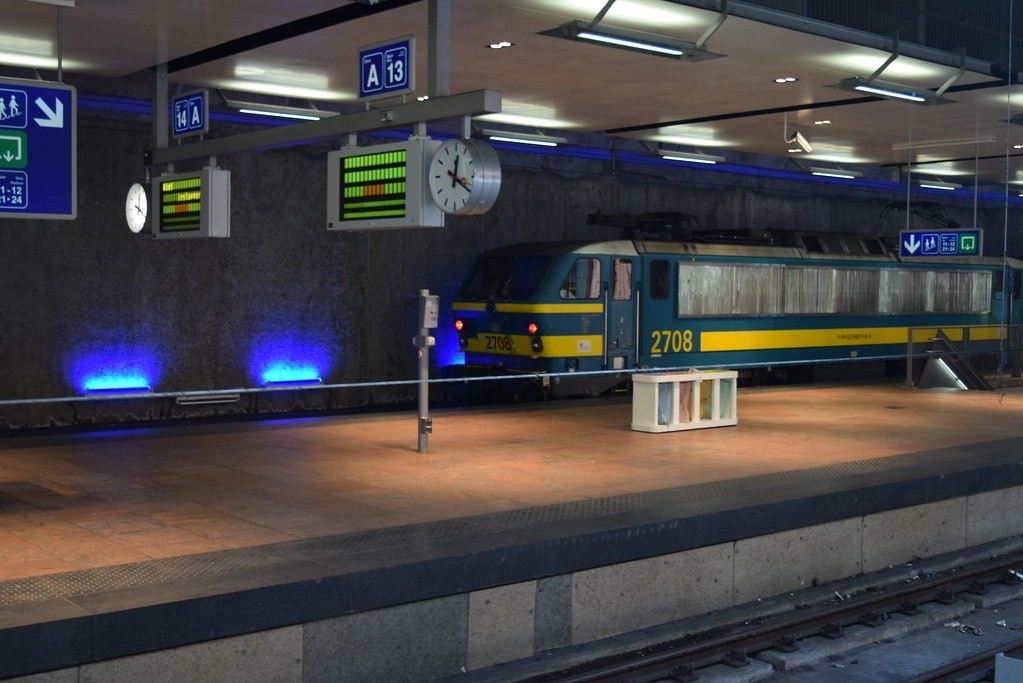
<box><xmin>451</xmin><ymin>226</ymin><xmax>1022</xmax><ymax>398</ymax></box>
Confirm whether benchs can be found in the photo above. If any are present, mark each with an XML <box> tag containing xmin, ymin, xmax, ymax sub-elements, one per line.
<box><xmin>631</xmin><ymin>370</ymin><xmax>740</xmax><ymax>434</ymax></box>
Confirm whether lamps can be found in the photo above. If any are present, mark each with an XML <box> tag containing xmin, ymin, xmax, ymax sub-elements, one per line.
<box><xmin>534</xmin><ymin>0</ymin><xmax>732</xmax><ymax>64</ymax></box>
<box><xmin>822</xmin><ymin>29</ymin><xmax>968</xmax><ymax>106</ymax></box>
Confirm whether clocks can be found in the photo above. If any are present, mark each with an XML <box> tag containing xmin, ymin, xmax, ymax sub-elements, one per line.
<box><xmin>428</xmin><ymin>113</ymin><xmax>502</xmax><ymax>217</ymax></box>
<box><xmin>123</xmin><ymin>181</ymin><xmax>151</xmax><ymax>235</ymax></box>
